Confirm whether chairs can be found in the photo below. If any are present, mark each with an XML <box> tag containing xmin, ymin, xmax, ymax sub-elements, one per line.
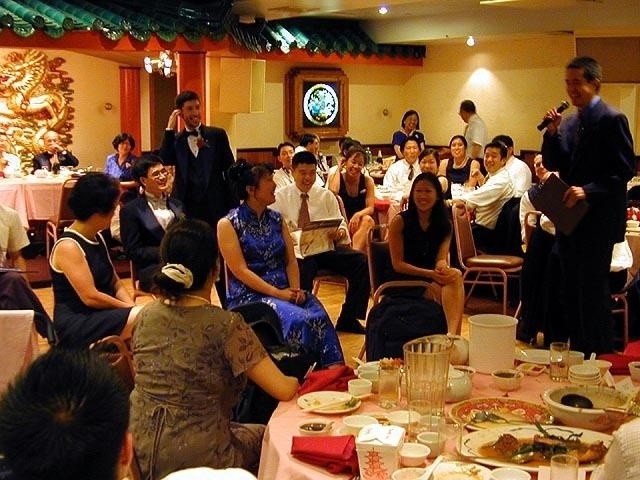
<box><xmin>46</xmin><ymin>179</ymin><xmax>80</xmax><ymax>260</ymax></box>
<box><xmin>451</xmin><ymin>199</ymin><xmax>525</xmax><ymax>316</ymax></box>
<box><xmin>119</xmin><ymin>191</ymin><xmax>176</xmax><ymax>306</ymax></box>
<box><xmin>334</xmin><ymin>194</ymin><xmax>349</xmax><ymax>225</ymax></box>
<box><xmin>223</xmin><ymin>258</ymin><xmax>319</xmax><ymax>390</ymax></box>
<box><xmin>311</xmin><ymin>274</ymin><xmax>350</xmax><ymax>299</ymax></box>
<box><xmin>89</xmin><ymin>334</ymin><xmax>137</xmax><ymax>405</ymax></box>
<box><xmin>524</xmin><ymin>211</ymin><xmax>557</xmax><ymax>254</ymax></box>
<box><xmin>358</xmin><ymin>224</ymin><xmax>441</xmax><ymax>360</ymax></box>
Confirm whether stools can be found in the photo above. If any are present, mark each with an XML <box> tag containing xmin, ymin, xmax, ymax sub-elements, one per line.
<box><xmin>607</xmin><ymin>290</ymin><xmax>629</xmax><ymax>348</ymax></box>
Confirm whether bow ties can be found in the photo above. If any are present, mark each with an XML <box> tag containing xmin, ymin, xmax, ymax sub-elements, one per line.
<box><xmin>185</xmin><ymin>130</ymin><xmax>198</xmax><ymax>137</ymax></box>
<box><xmin>146</xmin><ymin>196</ymin><xmax>168</xmax><ymax>211</ymax></box>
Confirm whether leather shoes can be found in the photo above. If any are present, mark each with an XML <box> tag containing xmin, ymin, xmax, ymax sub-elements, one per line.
<box><xmin>336</xmin><ymin>320</ymin><xmax>366</xmax><ymax>334</ymax></box>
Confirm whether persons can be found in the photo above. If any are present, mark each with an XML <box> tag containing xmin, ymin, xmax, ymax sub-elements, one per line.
<box><xmin>33</xmin><ymin>130</ymin><xmax>79</xmax><ymax>172</ymax></box>
<box><xmin>1</xmin><ymin>131</ymin><xmax>21</xmax><ymax>176</ymax></box>
<box><xmin>105</xmin><ymin>132</ymin><xmax>137</xmax><ymax>180</ymax></box>
<box><xmin>605</xmin><ymin>415</ymin><xmax>638</xmax><ymax>477</ymax></box>
<box><xmin>158</xmin><ymin>90</ymin><xmax>239</xmax><ymax>218</ymax></box>
<box><xmin>218</xmin><ymin>158</ymin><xmax>345</xmax><ymax>370</ymax></box>
<box><xmin>49</xmin><ymin>171</ymin><xmax>144</xmax><ymax>340</ymax></box>
<box><xmin>119</xmin><ymin>157</ymin><xmax>189</xmax><ymax>293</ymax></box>
<box><xmin>129</xmin><ymin>218</ymin><xmax>298</xmax><ymax>479</ymax></box>
<box><xmin>542</xmin><ymin>56</ymin><xmax>635</xmax><ymax>359</ymax></box>
<box><xmin>269</xmin><ymin>99</ymin><xmax>532</xmax><ymax>337</ymax></box>
<box><xmin>1</xmin><ymin>346</ymin><xmax>255</xmax><ymax>480</ymax></box>
<box><xmin>519</xmin><ymin>152</ymin><xmax>633</xmax><ymax>337</ymax></box>
<box><xmin>0</xmin><ymin>203</ymin><xmax>53</xmax><ymax>338</ymax></box>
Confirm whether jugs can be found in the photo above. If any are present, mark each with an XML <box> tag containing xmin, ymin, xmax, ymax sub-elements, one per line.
<box><xmin>399</xmin><ymin>334</ymin><xmax>455</xmax><ymax>457</ymax></box>
<box><xmin>445</xmin><ymin>368</ymin><xmax>471</xmax><ymax>405</ymax></box>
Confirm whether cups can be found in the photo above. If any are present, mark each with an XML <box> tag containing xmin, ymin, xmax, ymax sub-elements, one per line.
<box><xmin>549</xmin><ymin>343</ymin><xmax>570</xmax><ymax>382</ymax></box>
<box><xmin>548</xmin><ymin>454</ymin><xmax>580</xmax><ymax>480</ymax></box>
<box><xmin>378</xmin><ymin>366</ymin><xmax>398</xmax><ymax>410</ymax></box>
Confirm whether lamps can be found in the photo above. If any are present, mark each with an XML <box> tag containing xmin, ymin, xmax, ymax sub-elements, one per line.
<box><xmin>143</xmin><ymin>49</ymin><xmax>176</xmax><ymax>81</ymax></box>
<box><xmin>466</xmin><ymin>35</ymin><xmax>475</xmax><ymax>47</ymax></box>
<box><xmin>378</xmin><ymin>7</ymin><xmax>388</xmax><ymax>15</ymax></box>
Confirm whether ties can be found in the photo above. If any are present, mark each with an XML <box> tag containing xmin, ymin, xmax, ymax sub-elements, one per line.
<box><xmin>408</xmin><ymin>164</ymin><xmax>414</xmax><ymax>180</ymax></box>
<box><xmin>573</xmin><ymin>112</ymin><xmax>580</xmax><ymax>151</ymax></box>
<box><xmin>297</xmin><ymin>194</ymin><xmax>311</xmax><ymax>231</ymax></box>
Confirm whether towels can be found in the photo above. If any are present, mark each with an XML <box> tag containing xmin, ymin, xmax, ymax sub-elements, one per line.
<box><xmin>595</xmin><ymin>353</ymin><xmax>640</xmax><ymax>376</ymax></box>
<box><xmin>297</xmin><ymin>363</ymin><xmax>358</xmax><ymax>397</ymax></box>
<box><xmin>290</xmin><ymin>434</ymin><xmax>361</xmax><ymax>480</ymax></box>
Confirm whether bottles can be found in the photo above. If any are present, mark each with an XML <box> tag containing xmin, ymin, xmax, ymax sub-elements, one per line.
<box><xmin>376</xmin><ymin>149</ymin><xmax>383</xmax><ymax>164</ymax></box>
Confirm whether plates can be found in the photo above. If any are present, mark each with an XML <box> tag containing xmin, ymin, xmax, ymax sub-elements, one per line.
<box><xmin>448</xmin><ymin>395</ymin><xmax>555</xmax><ymax>432</ymax></box>
<box><xmin>296</xmin><ymin>391</ymin><xmax>362</xmax><ymax>417</ymax></box>
<box><xmin>457</xmin><ymin>425</ymin><xmax>613</xmax><ymax>472</ymax></box>
<box><xmin>513</xmin><ymin>349</ymin><xmax>564</xmax><ymax>363</ymax></box>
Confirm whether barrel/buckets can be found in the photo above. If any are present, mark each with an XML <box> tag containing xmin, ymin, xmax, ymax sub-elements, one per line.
<box><xmin>467</xmin><ymin>315</ymin><xmax>518</xmax><ymax>373</ymax></box>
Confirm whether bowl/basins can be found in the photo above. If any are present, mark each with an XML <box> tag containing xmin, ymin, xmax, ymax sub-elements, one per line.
<box><xmin>400</xmin><ymin>443</ymin><xmax>432</xmax><ymax>466</ymax></box>
<box><xmin>568</xmin><ymin>363</ymin><xmax>604</xmax><ymax>386</ymax></box>
<box><xmin>417</xmin><ymin>433</ymin><xmax>447</xmax><ymax>455</ymax></box>
<box><xmin>347</xmin><ymin>379</ymin><xmax>373</xmax><ymax>396</ymax></box>
<box><xmin>430</xmin><ymin>460</ymin><xmax>491</xmax><ymax>479</ymax></box>
<box><xmin>541</xmin><ymin>385</ymin><xmax>637</xmax><ymax>432</ymax></box>
<box><xmin>299</xmin><ymin>421</ymin><xmax>335</xmax><ymax>437</ymax></box>
<box><xmin>586</xmin><ymin>358</ymin><xmax>613</xmax><ymax>373</ymax></box>
<box><xmin>385</xmin><ymin>409</ymin><xmax>422</xmax><ymax>430</ymax></box>
<box><xmin>341</xmin><ymin>414</ymin><xmax>379</xmax><ymax>432</ymax></box>
<box><xmin>491</xmin><ymin>368</ymin><xmax>525</xmax><ymax>391</ymax></box>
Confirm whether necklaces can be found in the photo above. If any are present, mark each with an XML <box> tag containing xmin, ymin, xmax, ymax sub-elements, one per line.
<box><xmin>173</xmin><ymin>292</ymin><xmax>208</xmax><ymax>304</ymax></box>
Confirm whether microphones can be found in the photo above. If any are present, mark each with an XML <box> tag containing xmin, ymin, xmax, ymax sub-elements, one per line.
<box><xmin>537</xmin><ymin>100</ymin><xmax>570</xmax><ymax>132</ymax></box>
